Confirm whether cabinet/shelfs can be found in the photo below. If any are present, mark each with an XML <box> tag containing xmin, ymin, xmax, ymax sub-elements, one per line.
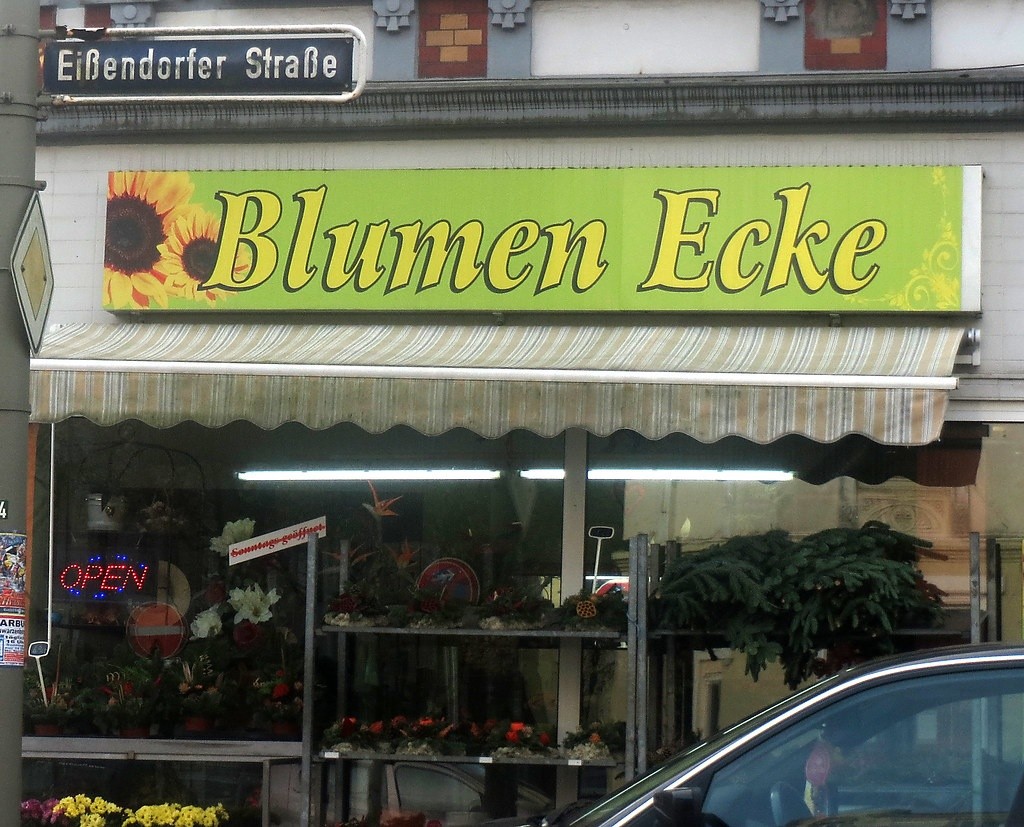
<box><xmin>639</xmin><ymin>531</ymin><xmax>1001</xmax><ymax>826</ymax></box>
<box><xmin>321</xmin><ymin>537</ymin><xmax>660</xmax><ymax>827</ymax></box>
<box><xmin>23</xmin><ymin>534</ymin><xmax>353</xmax><ymax>827</ymax></box>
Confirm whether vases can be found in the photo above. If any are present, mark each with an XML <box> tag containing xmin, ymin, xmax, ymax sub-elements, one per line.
<box><xmin>122</xmin><ymin>727</ymin><xmax>149</xmax><ymax>739</ymax></box>
<box><xmin>34</xmin><ymin>725</ymin><xmax>62</xmax><ymax>736</ymax></box>
<box><xmin>181</xmin><ymin>717</ymin><xmax>213</xmax><ymax>734</ymax></box>
<box><xmin>89</xmin><ymin>493</ymin><xmax>126</xmax><ymax>530</ymax></box>
<box><xmin>264</xmin><ymin>720</ymin><xmax>298</xmax><ymax>737</ymax></box>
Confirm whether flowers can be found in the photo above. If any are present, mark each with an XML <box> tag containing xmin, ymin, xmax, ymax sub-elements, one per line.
<box><xmin>22</xmin><ymin>500</ymin><xmax>327</xmax><ymax>827</ymax></box>
<box><xmin>325</xmin><ymin>479</ymin><xmax>629</xmax><ymax>758</ymax></box>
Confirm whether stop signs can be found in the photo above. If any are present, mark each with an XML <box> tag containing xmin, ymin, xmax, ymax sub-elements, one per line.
<box><xmin>127</xmin><ymin>603</ymin><xmax>190</xmax><ymax>660</ymax></box>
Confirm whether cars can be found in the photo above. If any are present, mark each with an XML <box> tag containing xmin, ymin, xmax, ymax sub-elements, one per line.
<box><xmin>243</xmin><ymin>756</ymin><xmax>552</xmax><ymax>827</ymax></box>
<box><xmin>522</xmin><ymin>639</ymin><xmax>1024</xmax><ymax>827</ymax></box>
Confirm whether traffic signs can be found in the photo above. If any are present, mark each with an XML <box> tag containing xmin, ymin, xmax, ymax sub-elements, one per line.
<box><xmin>41</xmin><ymin>27</ymin><xmax>373</xmax><ymax>101</ymax></box>
